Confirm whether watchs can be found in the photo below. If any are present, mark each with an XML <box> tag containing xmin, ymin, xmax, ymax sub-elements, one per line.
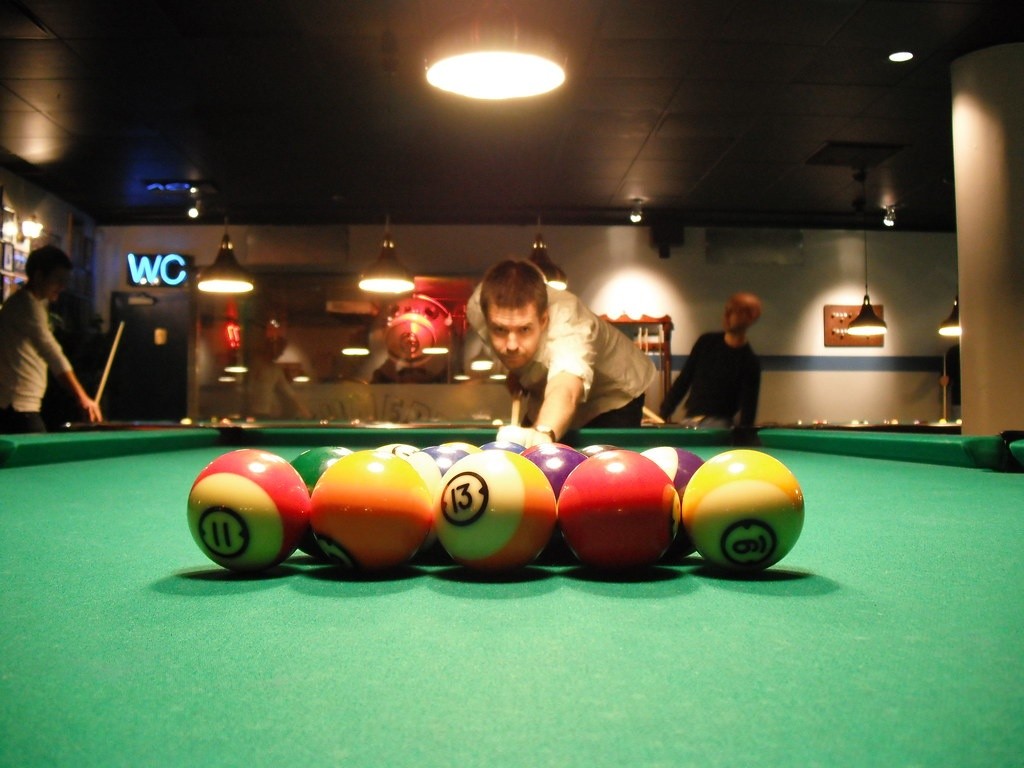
<box><xmin>532</xmin><ymin>424</ymin><xmax>557</xmax><ymax>444</ymax></box>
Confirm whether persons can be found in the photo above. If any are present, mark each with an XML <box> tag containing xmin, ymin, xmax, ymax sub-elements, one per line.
<box><xmin>642</xmin><ymin>293</ymin><xmax>765</xmax><ymax>430</ymax></box>
<box><xmin>0</xmin><ymin>244</ymin><xmax>104</xmax><ymax>433</ymax></box>
<box><xmin>939</xmin><ymin>343</ymin><xmax>961</xmax><ymax>423</ymax></box>
<box><xmin>466</xmin><ymin>258</ymin><xmax>658</xmax><ymax>449</ymax></box>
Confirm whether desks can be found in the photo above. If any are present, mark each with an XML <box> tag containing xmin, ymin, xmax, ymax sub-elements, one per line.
<box><xmin>0</xmin><ymin>424</ymin><xmax>1024</xmax><ymax>768</ymax></box>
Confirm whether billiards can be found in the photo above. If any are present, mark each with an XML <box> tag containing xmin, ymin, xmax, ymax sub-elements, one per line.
<box><xmin>683</xmin><ymin>449</ymin><xmax>805</xmax><ymax>572</ymax></box>
<box><xmin>558</xmin><ymin>450</ymin><xmax>681</xmax><ymax>572</ymax></box>
<box><xmin>811</xmin><ymin>416</ymin><xmax>962</xmax><ymax>425</ymax></box>
<box><xmin>310</xmin><ymin>450</ymin><xmax>434</xmax><ymax>574</ymax></box>
<box><xmin>187</xmin><ymin>449</ymin><xmax>310</xmax><ymax>573</ymax></box>
<box><xmin>435</xmin><ymin>450</ymin><xmax>558</xmax><ymax>573</ymax></box>
<box><xmin>290</xmin><ymin>440</ymin><xmax>706</xmax><ymax>560</ymax></box>
<box><xmin>177</xmin><ymin>414</ymin><xmax>504</xmax><ymax>428</ymax></box>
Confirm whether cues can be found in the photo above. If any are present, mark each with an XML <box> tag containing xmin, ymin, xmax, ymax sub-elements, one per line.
<box><xmin>636</xmin><ymin>322</ymin><xmax>666</xmax><ymax>424</ymax></box>
<box><xmin>511</xmin><ymin>389</ymin><xmax>521</xmax><ymax>427</ymax></box>
<box><xmin>93</xmin><ymin>321</ymin><xmax>125</xmax><ymax>402</ymax></box>
<box><xmin>942</xmin><ymin>350</ymin><xmax>947</xmax><ymax>420</ymax></box>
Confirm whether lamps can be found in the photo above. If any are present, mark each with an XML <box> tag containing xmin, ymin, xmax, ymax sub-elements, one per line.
<box><xmin>190</xmin><ymin>216</ymin><xmax>258</xmax><ymax>299</ymax></box>
<box><xmin>847</xmin><ymin>171</ymin><xmax>889</xmax><ymax>335</ymax></box>
<box><xmin>14</xmin><ymin>212</ymin><xmax>46</xmax><ymax>248</ymax></box>
<box><xmin>936</xmin><ymin>294</ymin><xmax>960</xmax><ymax>339</ymax></box>
<box><xmin>882</xmin><ymin>200</ymin><xmax>899</xmax><ymax>227</ymax></box>
<box><xmin>185</xmin><ymin>201</ymin><xmax>204</xmax><ymax>222</ymax></box>
<box><xmin>357</xmin><ymin>213</ymin><xmax>416</xmax><ymax>293</ymax></box>
<box><xmin>524</xmin><ymin>214</ymin><xmax>568</xmax><ymax>289</ymax></box>
<box><xmin>628</xmin><ymin>198</ymin><xmax>646</xmax><ymax>225</ymax></box>
